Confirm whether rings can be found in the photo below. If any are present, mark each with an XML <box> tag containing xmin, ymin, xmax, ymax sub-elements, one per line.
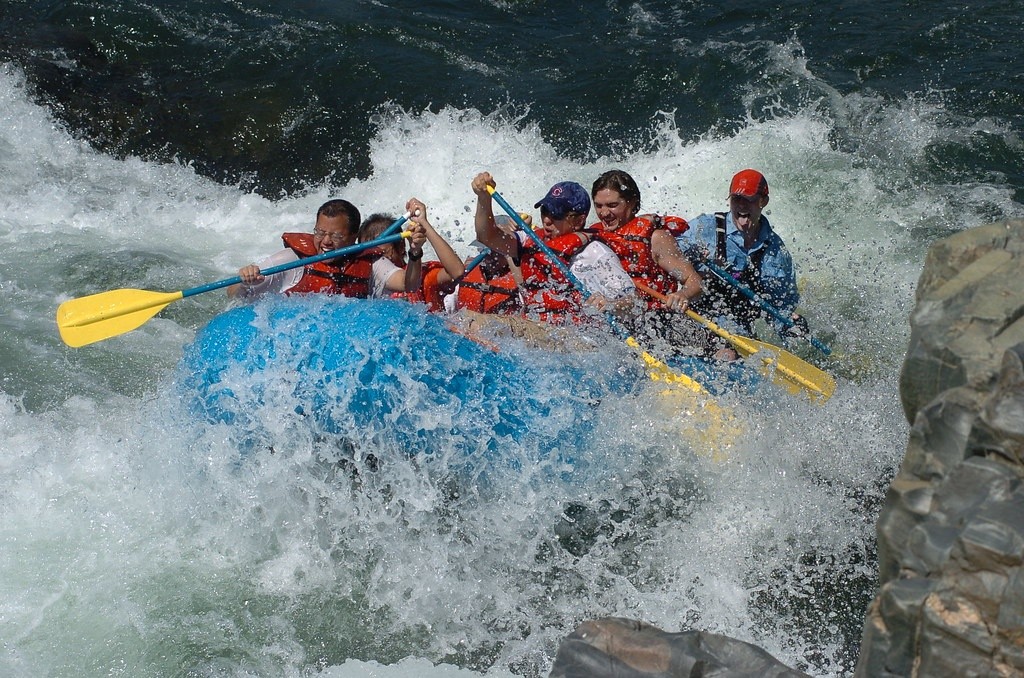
<box><xmin>679</xmin><ymin>303</ymin><xmax>683</xmax><ymax>307</ymax></box>
<box><xmin>683</xmin><ymin>300</ymin><xmax>689</xmax><ymax>304</ymax></box>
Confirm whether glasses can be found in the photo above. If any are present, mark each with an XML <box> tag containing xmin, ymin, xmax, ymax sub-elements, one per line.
<box><xmin>313</xmin><ymin>227</ymin><xmax>351</xmax><ymax>244</ymax></box>
<box><xmin>540</xmin><ymin>204</ymin><xmax>579</xmax><ymax>221</ymax></box>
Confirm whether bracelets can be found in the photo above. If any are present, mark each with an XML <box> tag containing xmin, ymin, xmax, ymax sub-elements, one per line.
<box><xmin>408</xmin><ymin>249</ymin><xmax>424</xmax><ymax>262</ymax></box>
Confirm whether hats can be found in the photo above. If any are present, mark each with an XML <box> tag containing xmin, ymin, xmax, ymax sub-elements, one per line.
<box><xmin>468</xmin><ymin>215</ymin><xmax>511</xmax><ymax>249</ymax></box>
<box><xmin>534</xmin><ymin>181</ymin><xmax>591</xmax><ymax>218</ymax></box>
<box><xmin>725</xmin><ymin>169</ymin><xmax>770</xmax><ymax>202</ymax></box>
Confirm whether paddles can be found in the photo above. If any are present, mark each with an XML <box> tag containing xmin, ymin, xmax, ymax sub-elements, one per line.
<box><xmin>445</xmin><ymin>213</ymin><xmax>528</xmax><ymax>298</ymax></box>
<box><xmin>485</xmin><ymin>184</ymin><xmax>743</xmax><ymax>462</ymax></box>
<box><xmin>56</xmin><ymin>222</ymin><xmax>419</xmax><ymax>349</ymax></box>
<box><xmin>700</xmin><ymin>255</ymin><xmax>867</xmax><ymax>382</ymax></box>
<box><xmin>520</xmin><ymin>212</ymin><xmax>835</xmax><ymax>407</ymax></box>
<box><xmin>370</xmin><ymin>201</ymin><xmax>420</xmax><ymax>247</ymax></box>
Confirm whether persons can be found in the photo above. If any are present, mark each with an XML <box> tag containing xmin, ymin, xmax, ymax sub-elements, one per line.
<box><xmin>224</xmin><ymin>170</ymin><xmax>809</xmax><ymax>363</ymax></box>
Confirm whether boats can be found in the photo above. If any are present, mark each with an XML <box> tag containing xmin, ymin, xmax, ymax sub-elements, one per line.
<box><xmin>175</xmin><ymin>289</ymin><xmax>831</xmax><ymax>503</ymax></box>
